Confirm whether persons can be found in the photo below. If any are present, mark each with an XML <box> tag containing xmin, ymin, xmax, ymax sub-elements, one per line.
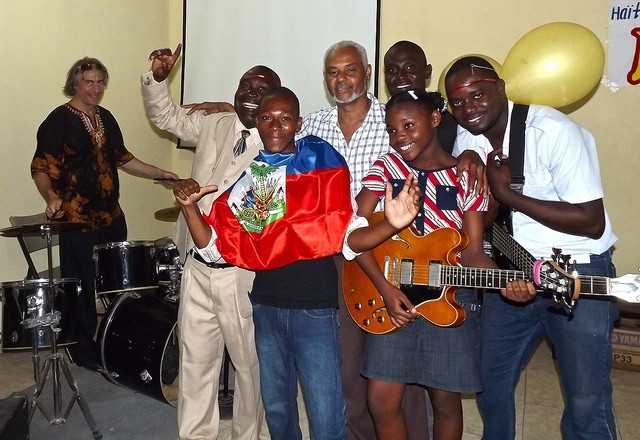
<box><xmin>31</xmin><ymin>57</ymin><xmax>182</xmax><ymax>375</ymax></box>
<box><xmin>382</xmin><ymin>40</ymin><xmax>432</xmax><ymax>98</ymax></box>
<box><xmin>349</xmin><ymin>90</ymin><xmax>486</xmax><ymax>439</ymax></box>
<box><xmin>218</xmin><ymin>87</ymin><xmax>349</xmax><ymax>439</ymax></box>
<box><xmin>303</xmin><ymin>40</ymin><xmax>383</xmax><ymax>157</ymax></box>
<box><xmin>448</xmin><ymin>58</ymin><xmax>621</xmax><ymax>438</ymax></box>
<box><xmin>144</xmin><ymin>44</ymin><xmax>280</xmax><ymax>439</ymax></box>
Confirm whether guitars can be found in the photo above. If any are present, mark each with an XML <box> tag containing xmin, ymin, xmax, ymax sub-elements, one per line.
<box><xmin>456</xmin><ymin>149</ymin><xmax>582</xmax><ymax>321</ymax></box>
<box><xmin>341</xmin><ymin>210</ymin><xmax>639</xmax><ymax>335</ymax></box>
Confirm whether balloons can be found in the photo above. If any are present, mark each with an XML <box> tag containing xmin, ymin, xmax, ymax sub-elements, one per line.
<box><xmin>437</xmin><ymin>50</ymin><xmax>502</xmax><ymax>104</ymax></box>
<box><xmin>500</xmin><ymin>19</ymin><xmax>607</xmax><ymax>108</ymax></box>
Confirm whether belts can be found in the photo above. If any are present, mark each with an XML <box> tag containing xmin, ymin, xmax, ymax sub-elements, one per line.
<box><xmin>190</xmin><ymin>250</ymin><xmax>238</xmax><ymax>269</ymax></box>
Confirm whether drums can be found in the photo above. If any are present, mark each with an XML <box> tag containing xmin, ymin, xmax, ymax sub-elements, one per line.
<box><xmin>92</xmin><ymin>241</ymin><xmax>160</xmax><ymax>295</ymax></box>
<box><xmin>0</xmin><ymin>278</ymin><xmax>82</xmax><ymax>350</ymax></box>
<box><xmin>95</xmin><ymin>289</ymin><xmax>179</xmax><ymax>409</ymax></box>
<box><xmin>155</xmin><ymin>236</ymin><xmax>183</xmax><ymax>288</ymax></box>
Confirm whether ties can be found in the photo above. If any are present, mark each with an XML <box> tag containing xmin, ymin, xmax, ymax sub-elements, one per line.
<box><xmin>233</xmin><ymin>130</ymin><xmax>250</xmax><ymax>158</ymax></box>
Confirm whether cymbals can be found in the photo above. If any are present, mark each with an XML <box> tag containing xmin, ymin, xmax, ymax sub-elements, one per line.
<box><xmin>1</xmin><ymin>222</ymin><xmax>93</xmax><ymax>237</ymax></box>
<box><xmin>154</xmin><ymin>206</ymin><xmax>182</xmax><ymax>222</ymax></box>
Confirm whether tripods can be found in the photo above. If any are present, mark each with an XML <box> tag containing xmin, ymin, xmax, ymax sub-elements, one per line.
<box><xmin>28</xmin><ymin>234</ymin><xmax>103</xmax><ymax>440</ymax></box>
